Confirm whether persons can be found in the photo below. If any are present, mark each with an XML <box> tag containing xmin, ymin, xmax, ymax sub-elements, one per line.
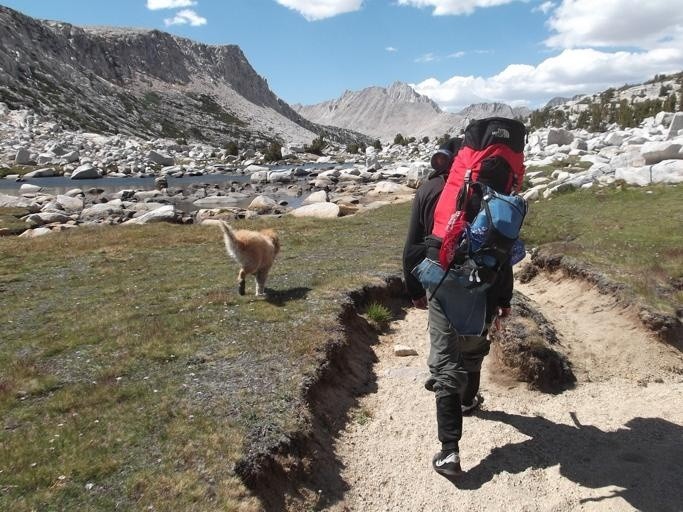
<box><xmin>402</xmin><ymin>136</ymin><xmax>514</xmax><ymax>476</ymax></box>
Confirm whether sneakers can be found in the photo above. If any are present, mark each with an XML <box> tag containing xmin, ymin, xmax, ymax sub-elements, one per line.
<box><xmin>433</xmin><ymin>450</ymin><xmax>463</xmax><ymax>476</ymax></box>
<box><xmin>462</xmin><ymin>389</ymin><xmax>481</xmax><ymax>414</ymax></box>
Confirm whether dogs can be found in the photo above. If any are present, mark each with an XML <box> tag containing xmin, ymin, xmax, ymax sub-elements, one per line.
<box><xmin>218</xmin><ymin>219</ymin><xmax>281</xmax><ymax>297</ymax></box>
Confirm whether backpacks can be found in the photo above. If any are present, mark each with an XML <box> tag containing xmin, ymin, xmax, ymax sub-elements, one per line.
<box><xmin>416</xmin><ymin>118</ymin><xmax>528</xmax><ymax>284</ymax></box>
